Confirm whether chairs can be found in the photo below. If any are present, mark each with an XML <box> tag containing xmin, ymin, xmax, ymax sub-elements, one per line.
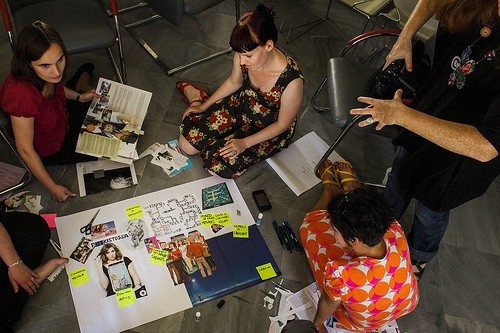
<box><xmin>278</xmin><ymin>0</ymin><xmax>400</xmax><ymax>65</ymax></box>
<box><xmin>0</xmin><ymin>0</ymin><xmax>129</xmax><ymax>85</ymax></box>
<box><xmin>104</xmin><ymin>0</ymin><xmax>241</xmax><ymax>74</ymax></box>
<box><xmin>311</xmin><ymin>28</ymin><xmax>430</xmax><ymax>181</ymax></box>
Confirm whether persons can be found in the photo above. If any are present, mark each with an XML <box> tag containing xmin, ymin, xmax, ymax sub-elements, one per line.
<box><xmin>0</xmin><ymin>20</ymin><xmax>103</xmax><ymax>202</ymax></box>
<box><xmin>300</xmin><ymin>160</ymin><xmax>420</xmax><ymax>333</ymax></box>
<box><xmin>211</xmin><ymin>224</ymin><xmax>221</xmax><ymax>233</ymax></box>
<box><xmin>350</xmin><ymin>0</ymin><xmax>500</xmax><ymax>282</ymax></box>
<box><xmin>176</xmin><ymin>4</ymin><xmax>305</xmax><ymax>179</ymax></box>
<box><xmin>0</xmin><ymin>211</ymin><xmax>69</xmax><ymax>333</ymax></box>
<box><xmin>73</xmin><ymin>226</ymin><xmax>141</xmax><ymax>297</ymax></box>
<box><xmin>81</xmin><ymin>85</ymin><xmax>137</xmax><ymax>143</ymax></box>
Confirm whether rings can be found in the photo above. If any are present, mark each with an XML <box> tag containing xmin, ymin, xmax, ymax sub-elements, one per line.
<box><xmin>234</xmin><ymin>151</ymin><xmax>237</xmax><ymax>154</ymax></box>
<box><xmin>366</xmin><ymin>118</ymin><xmax>373</xmax><ymax>124</ymax></box>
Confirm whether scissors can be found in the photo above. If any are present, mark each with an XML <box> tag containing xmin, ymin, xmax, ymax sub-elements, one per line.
<box><xmin>79</xmin><ymin>208</ymin><xmax>101</xmax><ymax>236</ymax></box>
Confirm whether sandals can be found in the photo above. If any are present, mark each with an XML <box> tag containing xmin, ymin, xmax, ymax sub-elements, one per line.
<box><xmin>334</xmin><ymin>161</ymin><xmax>361</xmax><ymax>193</ymax></box>
<box><xmin>319</xmin><ymin>159</ymin><xmax>342</xmax><ymax>192</ymax></box>
<box><xmin>175</xmin><ymin>80</ymin><xmax>211</xmax><ymax>105</ymax></box>
<box><xmin>410</xmin><ymin>258</ymin><xmax>426</xmax><ymax>282</ymax></box>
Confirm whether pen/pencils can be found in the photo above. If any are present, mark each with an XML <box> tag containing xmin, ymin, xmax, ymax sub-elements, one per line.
<box><xmin>270</xmin><ymin>219</ymin><xmax>303</xmax><ymax>255</ymax></box>
<box><xmin>271</xmin><ymin>281</ymin><xmax>296</xmax><ymax>297</ymax></box>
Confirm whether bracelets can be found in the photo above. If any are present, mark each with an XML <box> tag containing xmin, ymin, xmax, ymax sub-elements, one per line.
<box><xmin>7</xmin><ymin>260</ymin><xmax>23</xmax><ymax>269</ymax></box>
<box><xmin>77</xmin><ymin>92</ymin><xmax>81</xmax><ymax>102</ymax></box>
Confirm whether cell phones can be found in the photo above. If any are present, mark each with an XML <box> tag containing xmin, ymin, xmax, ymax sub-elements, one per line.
<box><xmin>252</xmin><ymin>190</ymin><xmax>272</xmax><ymax>211</ymax></box>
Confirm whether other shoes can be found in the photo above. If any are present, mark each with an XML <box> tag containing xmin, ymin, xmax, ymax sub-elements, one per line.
<box><xmin>66</xmin><ymin>63</ymin><xmax>95</xmax><ymax>93</ymax></box>
